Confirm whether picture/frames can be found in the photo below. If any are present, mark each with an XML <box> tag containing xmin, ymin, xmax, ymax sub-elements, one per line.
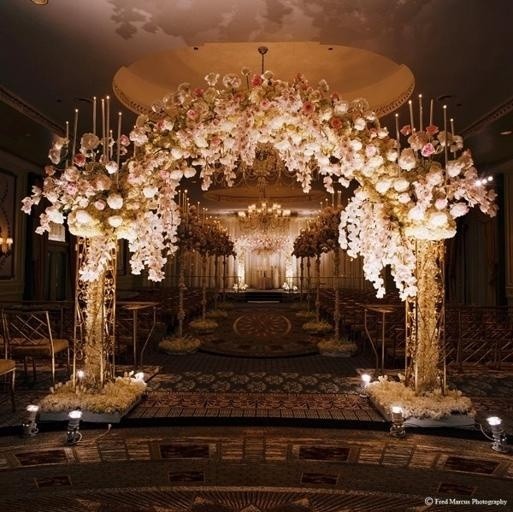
<box><xmin>0</xmin><ymin>166</ymin><xmax>19</xmax><ymax>284</ymax></box>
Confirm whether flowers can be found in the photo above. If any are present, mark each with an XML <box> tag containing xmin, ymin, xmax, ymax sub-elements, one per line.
<box><xmin>23</xmin><ymin>66</ymin><xmax>498</xmax><ymax>421</ymax></box>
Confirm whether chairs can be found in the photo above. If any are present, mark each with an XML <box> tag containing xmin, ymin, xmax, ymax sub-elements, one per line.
<box><xmin>0</xmin><ymin>287</ymin><xmax>211</xmax><ymax>412</ymax></box>
<box><xmin>313</xmin><ymin>286</ymin><xmax>513</xmax><ymax>371</ymax></box>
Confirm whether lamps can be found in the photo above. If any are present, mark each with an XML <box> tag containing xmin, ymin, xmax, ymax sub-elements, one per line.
<box><xmin>209</xmin><ymin>46</ymin><xmax>319</xmax><ymax>257</ymax></box>
